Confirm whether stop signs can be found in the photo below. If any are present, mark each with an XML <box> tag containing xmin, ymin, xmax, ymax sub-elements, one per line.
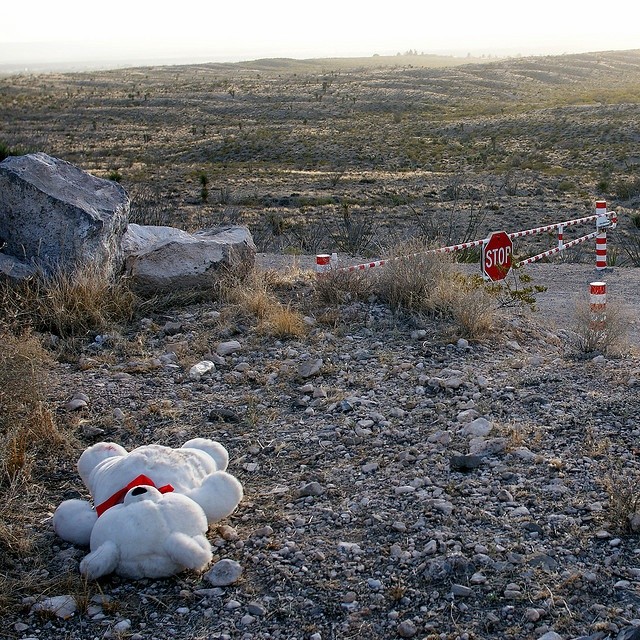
<box><xmin>481</xmin><ymin>230</ymin><xmax>512</xmax><ymax>283</ymax></box>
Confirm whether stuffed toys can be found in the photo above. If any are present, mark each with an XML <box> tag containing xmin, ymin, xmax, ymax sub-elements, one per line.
<box><xmin>53</xmin><ymin>438</ymin><xmax>243</xmax><ymax>579</ymax></box>
